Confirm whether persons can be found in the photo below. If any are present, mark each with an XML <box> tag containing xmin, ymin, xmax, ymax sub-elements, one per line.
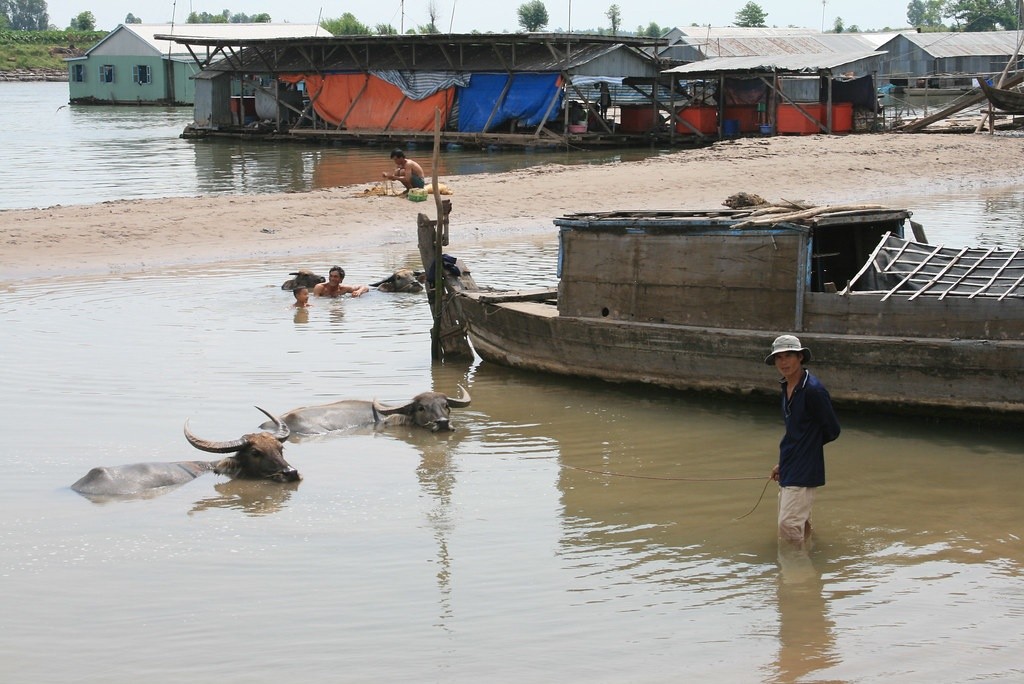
<box><xmin>595</xmin><ymin>83</ymin><xmax>611</xmax><ymax>120</ymax></box>
<box><xmin>293</xmin><ymin>286</ymin><xmax>311</xmax><ymax>307</ymax></box>
<box><xmin>763</xmin><ymin>335</ymin><xmax>841</xmax><ymax>547</ymax></box>
<box><xmin>313</xmin><ymin>265</ymin><xmax>369</xmax><ymax>298</ymax></box>
<box><xmin>382</xmin><ymin>148</ymin><xmax>424</xmax><ymax>196</ymax></box>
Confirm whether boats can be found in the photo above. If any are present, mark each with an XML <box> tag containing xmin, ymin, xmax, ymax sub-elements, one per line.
<box><xmin>414</xmin><ymin>199</ymin><xmax>1024</xmax><ymax>419</ymax></box>
<box><xmin>976</xmin><ymin>73</ymin><xmax>1024</xmax><ymax>114</ymax></box>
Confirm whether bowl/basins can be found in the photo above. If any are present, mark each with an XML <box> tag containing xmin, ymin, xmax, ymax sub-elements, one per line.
<box><xmin>568</xmin><ymin>125</ymin><xmax>587</xmax><ymax>133</ymax></box>
<box><xmin>760</xmin><ymin>126</ymin><xmax>773</xmax><ymax>134</ymax></box>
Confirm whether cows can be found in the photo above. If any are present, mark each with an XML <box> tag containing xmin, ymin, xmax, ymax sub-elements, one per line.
<box><xmin>281</xmin><ymin>269</ymin><xmax>326</xmax><ymax>290</ymax></box>
<box><xmin>368</xmin><ymin>267</ymin><xmax>427</xmax><ymax>293</ymax></box>
<box><xmin>68</xmin><ymin>405</ymin><xmax>301</xmax><ymax>498</ymax></box>
<box><xmin>258</xmin><ymin>384</ymin><xmax>472</xmax><ymax>435</ymax></box>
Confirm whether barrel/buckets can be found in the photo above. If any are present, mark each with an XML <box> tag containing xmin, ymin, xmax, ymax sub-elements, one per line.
<box><xmin>723</xmin><ymin>120</ymin><xmax>738</xmax><ymax>136</ymax></box>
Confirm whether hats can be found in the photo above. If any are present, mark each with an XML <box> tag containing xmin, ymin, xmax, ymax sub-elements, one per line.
<box><xmin>765</xmin><ymin>335</ymin><xmax>812</xmax><ymax>366</ymax></box>
